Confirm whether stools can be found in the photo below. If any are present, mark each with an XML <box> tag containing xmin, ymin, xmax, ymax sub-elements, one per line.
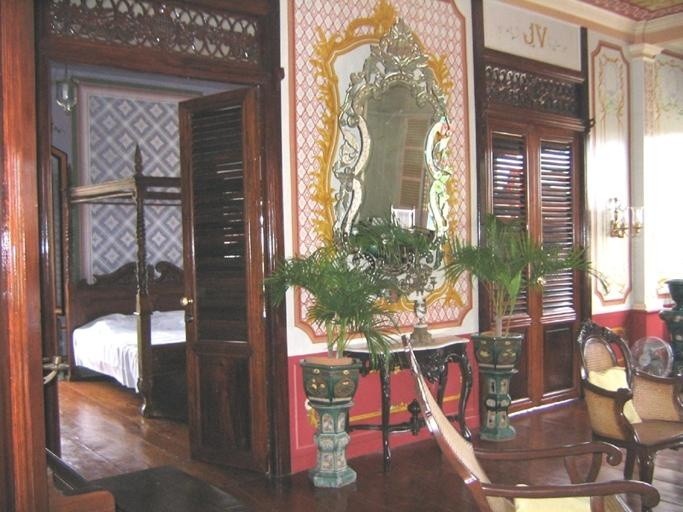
<box><xmin>88</xmin><ymin>466</ymin><xmax>241</xmax><ymax>512</ymax></box>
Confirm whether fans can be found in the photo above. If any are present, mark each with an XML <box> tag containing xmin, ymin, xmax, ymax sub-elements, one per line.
<box><xmin>630</xmin><ymin>336</ymin><xmax>674</xmax><ymax>378</ymax></box>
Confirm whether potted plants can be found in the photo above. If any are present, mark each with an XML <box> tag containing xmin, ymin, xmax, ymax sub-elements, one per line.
<box><xmin>438</xmin><ymin>213</ymin><xmax>609</xmax><ymax>371</ymax></box>
<box><xmin>256</xmin><ymin>208</ymin><xmax>434</xmax><ymax>405</ymax></box>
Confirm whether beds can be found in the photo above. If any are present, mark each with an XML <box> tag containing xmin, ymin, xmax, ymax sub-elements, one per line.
<box><xmin>65</xmin><ymin>144</ymin><xmax>187</xmax><ymax>417</ymax></box>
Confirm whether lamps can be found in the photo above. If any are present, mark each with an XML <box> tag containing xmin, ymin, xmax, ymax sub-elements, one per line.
<box><xmin>609</xmin><ymin>204</ymin><xmax>645</xmax><ymax>238</ymax></box>
<box><xmin>56</xmin><ymin>64</ymin><xmax>80</xmax><ymax>112</ymax></box>
<box><xmin>53</xmin><ymin>60</ymin><xmax>79</xmax><ymax>116</ymax></box>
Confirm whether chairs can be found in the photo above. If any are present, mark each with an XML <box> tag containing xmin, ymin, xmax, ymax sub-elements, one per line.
<box><xmin>577</xmin><ymin>318</ymin><xmax>683</xmax><ymax>512</ymax></box>
<box><xmin>402</xmin><ymin>335</ymin><xmax>660</xmax><ymax>512</ymax></box>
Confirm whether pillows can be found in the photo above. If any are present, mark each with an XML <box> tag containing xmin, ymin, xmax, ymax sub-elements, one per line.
<box><xmin>514</xmin><ymin>482</ymin><xmax>591</xmax><ymax>512</ymax></box>
<box><xmin>587</xmin><ymin>366</ymin><xmax>642</xmax><ymax>424</ymax></box>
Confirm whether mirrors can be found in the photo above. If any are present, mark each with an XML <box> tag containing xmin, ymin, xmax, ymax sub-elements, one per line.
<box><xmin>305</xmin><ymin>0</ymin><xmax>465</xmax><ymax>326</ymax></box>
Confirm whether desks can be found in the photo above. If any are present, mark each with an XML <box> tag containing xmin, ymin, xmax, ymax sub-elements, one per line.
<box><xmin>343</xmin><ymin>336</ymin><xmax>474</xmax><ymax>476</ymax></box>
